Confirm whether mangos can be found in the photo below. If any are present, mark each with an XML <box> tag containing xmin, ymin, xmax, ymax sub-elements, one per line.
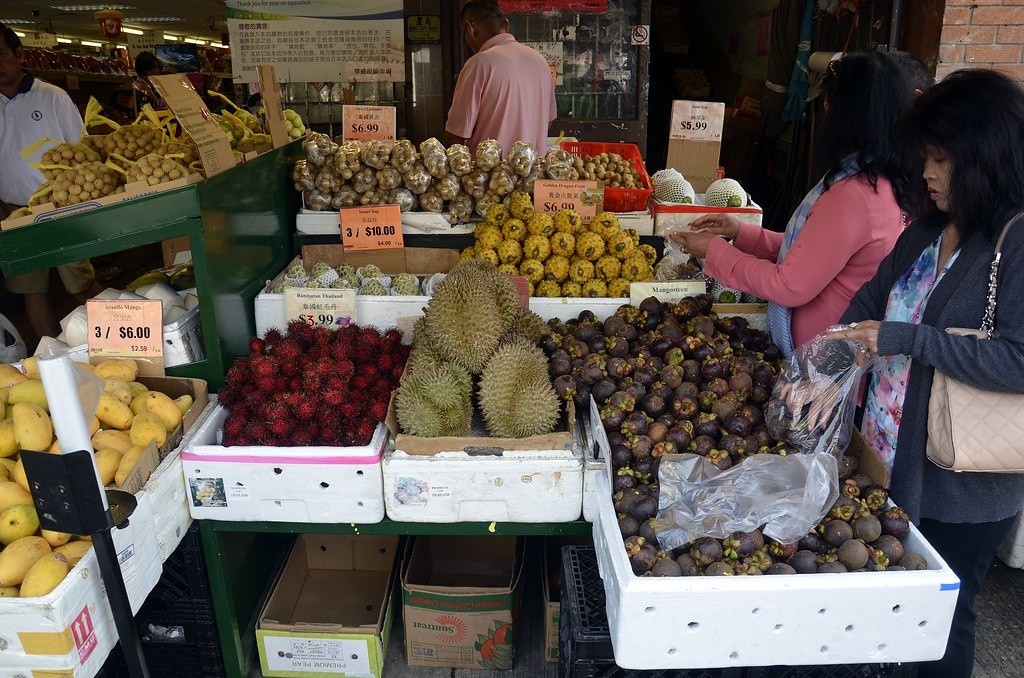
<box><xmin>122</xmin><ymin>266</ymin><xmax>195</xmax><ymax>292</ymax></box>
<box><xmin>0</xmin><ymin>354</ymin><xmax>194</xmax><ymax>597</ymax></box>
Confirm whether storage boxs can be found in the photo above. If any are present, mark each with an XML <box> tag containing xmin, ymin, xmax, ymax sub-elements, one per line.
<box><xmin>723</xmin><ymin>95</ymin><xmax>761</xmax><ymax>145</ymax></box>
<box><xmin>0</xmin><ymin>140</ymin><xmax>960</xmax><ymax>678</ymax></box>
<box><xmin>652</xmin><ymin>3</ymin><xmax>712</xmax><ymax>98</ymax></box>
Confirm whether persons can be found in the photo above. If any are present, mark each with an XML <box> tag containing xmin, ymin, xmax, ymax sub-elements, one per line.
<box><xmin>779</xmin><ymin>69</ymin><xmax>1024</xmax><ymax>678</ymax></box>
<box><xmin>676</xmin><ymin>50</ymin><xmax>920</xmax><ymax>359</ymax></box>
<box><xmin>135</xmin><ymin>51</ymin><xmax>168</xmax><ymax>111</ymax></box>
<box><xmin>0</xmin><ymin>21</ymin><xmax>103</xmax><ymax>338</ymax></box>
<box><xmin>888</xmin><ymin>51</ymin><xmax>931</xmax><ymax>112</ymax></box>
<box><xmin>445</xmin><ymin>0</ymin><xmax>556</xmax><ymax>159</ymax></box>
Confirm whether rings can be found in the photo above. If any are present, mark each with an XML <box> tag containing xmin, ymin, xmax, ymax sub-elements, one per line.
<box><xmin>710</xmin><ymin>221</ymin><xmax>714</xmax><ymax>227</ymax></box>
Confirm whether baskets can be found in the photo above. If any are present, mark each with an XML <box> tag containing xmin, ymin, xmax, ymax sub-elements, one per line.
<box><xmin>560</xmin><ymin>141</ymin><xmax>653</xmax><ymax>213</ymax></box>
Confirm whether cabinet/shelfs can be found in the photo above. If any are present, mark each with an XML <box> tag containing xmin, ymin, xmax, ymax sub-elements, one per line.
<box><xmin>20</xmin><ymin>69</ymin><xmax>142</xmax><ymax>136</ymax></box>
<box><xmin>197</xmin><ymin>520</ymin><xmax>598</xmax><ymax>677</ymax></box>
<box><xmin>0</xmin><ymin>135</ymin><xmax>307</xmax><ymax>390</ymax></box>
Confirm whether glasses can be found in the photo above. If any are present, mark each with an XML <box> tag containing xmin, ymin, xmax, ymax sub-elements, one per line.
<box><xmin>826</xmin><ymin>59</ymin><xmax>842</xmax><ymax>81</ymax></box>
<box><xmin>462</xmin><ymin>19</ymin><xmax>473</xmax><ymax>45</ymax></box>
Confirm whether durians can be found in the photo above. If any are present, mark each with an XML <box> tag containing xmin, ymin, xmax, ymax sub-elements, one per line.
<box><xmin>393</xmin><ymin>255</ymin><xmax>561</xmax><ymax>437</ymax></box>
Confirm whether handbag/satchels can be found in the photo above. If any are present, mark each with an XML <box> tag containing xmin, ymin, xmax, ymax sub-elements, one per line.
<box><xmin>926</xmin><ymin>211</ymin><xmax>1024</xmax><ymax>473</ymax></box>
